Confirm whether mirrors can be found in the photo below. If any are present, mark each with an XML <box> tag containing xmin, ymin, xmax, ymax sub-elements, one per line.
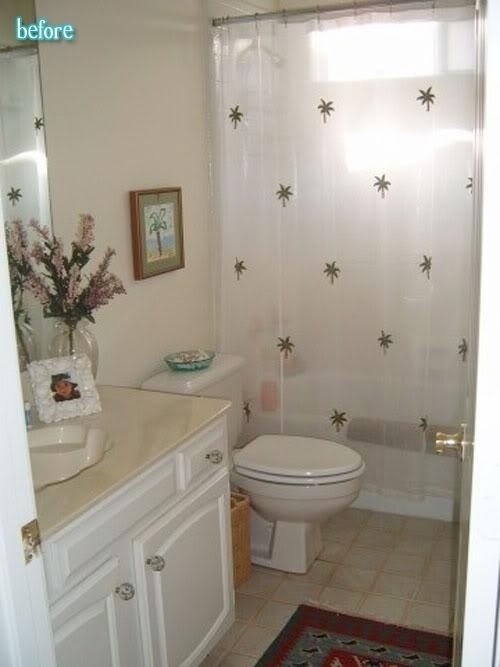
<box><xmin>0</xmin><ymin>4</ymin><xmax>54</xmax><ymax>406</ymax></box>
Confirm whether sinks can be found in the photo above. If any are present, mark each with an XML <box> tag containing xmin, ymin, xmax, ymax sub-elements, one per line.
<box><xmin>29</xmin><ymin>423</ymin><xmax>114</xmax><ymax>492</ymax></box>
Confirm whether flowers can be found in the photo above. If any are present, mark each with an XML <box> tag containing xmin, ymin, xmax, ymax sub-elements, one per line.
<box><xmin>4</xmin><ymin>217</ymin><xmax>37</xmax><ymax>324</ymax></box>
<box><xmin>24</xmin><ymin>214</ymin><xmax>127</xmax><ymax>352</ymax></box>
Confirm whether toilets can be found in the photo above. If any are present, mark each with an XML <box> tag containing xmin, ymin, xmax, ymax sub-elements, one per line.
<box><xmin>141</xmin><ymin>353</ymin><xmax>368</xmax><ymax>572</ymax></box>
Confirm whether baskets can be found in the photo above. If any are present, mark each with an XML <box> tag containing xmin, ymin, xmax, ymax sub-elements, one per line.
<box><xmin>230</xmin><ymin>490</ymin><xmax>251</xmax><ymax>589</ymax></box>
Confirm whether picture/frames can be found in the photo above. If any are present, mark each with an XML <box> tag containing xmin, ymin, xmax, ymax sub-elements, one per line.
<box><xmin>26</xmin><ymin>352</ymin><xmax>102</xmax><ymax>424</ymax></box>
<box><xmin>129</xmin><ymin>186</ymin><xmax>185</xmax><ymax>280</ymax></box>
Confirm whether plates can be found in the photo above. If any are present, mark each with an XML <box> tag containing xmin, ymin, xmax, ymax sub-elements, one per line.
<box><xmin>163</xmin><ymin>350</ymin><xmax>216</xmax><ymax>370</ymax></box>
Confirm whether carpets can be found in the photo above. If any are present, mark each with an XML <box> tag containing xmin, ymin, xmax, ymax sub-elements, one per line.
<box><xmin>254</xmin><ymin>604</ymin><xmax>453</xmax><ymax>667</ymax></box>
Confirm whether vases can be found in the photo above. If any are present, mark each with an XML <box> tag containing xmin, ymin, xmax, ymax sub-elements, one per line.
<box><xmin>39</xmin><ymin>321</ymin><xmax>98</xmax><ymax>379</ymax></box>
<box><xmin>14</xmin><ymin>312</ymin><xmax>37</xmax><ymax>371</ymax></box>
